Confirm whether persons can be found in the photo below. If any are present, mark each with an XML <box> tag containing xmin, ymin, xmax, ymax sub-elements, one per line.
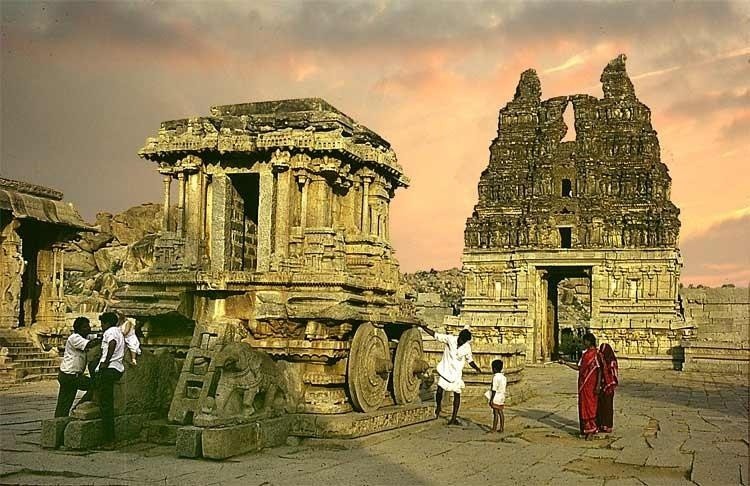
<box><xmin>577</xmin><ymin>340</ymin><xmax>585</xmax><ymax>359</ymax></box>
<box><xmin>599</xmin><ymin>343</ymin><xmax>618</xmax><ymax>433</ymax></box>
<box><xmin>116</xmin><ymin>311</ymin><xmax>141</xmax><ymax>365</ymax></box>
<box><xmin>421</xmin><ymin>322</ymin><xmax>482</xmax><ymax>426</ymax></box>
<box><xmin>489</xmin><ymin>359</ymin><xmax>508</xmax><ymax>432</ymax></box>
<box><xmin>450</xmin><ymin>300</ymin><xmax>460</xmax><ymax>317</ymax></box>
<box><xmin>52</xmin><ymin>317</ymin><xmax>100</xmax><ymax>418</ymax></box>
<box><xmin>553</xmin><ymin>334</ymin><xmax>603</xmax><ymax>440</ymax></box>
<box><xmin>87</xmin><ymin>311</ymin><xmax>125</xmax><ymax>450</ymax></box>
<box><xmin>570</xmin><ymin>340</ymin><xmax>577</xmax><ymax>360</ymax></box>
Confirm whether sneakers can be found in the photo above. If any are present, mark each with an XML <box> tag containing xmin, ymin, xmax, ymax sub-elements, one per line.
<box><xmin>130</xmin><ymin>359</ymin><xmax>137</xmax><ymax>365</ymax></box>
<box><xmin>95</xmin><ymin>441</ymin><xmax>114</xmax><ymax>450</ymax></box>
